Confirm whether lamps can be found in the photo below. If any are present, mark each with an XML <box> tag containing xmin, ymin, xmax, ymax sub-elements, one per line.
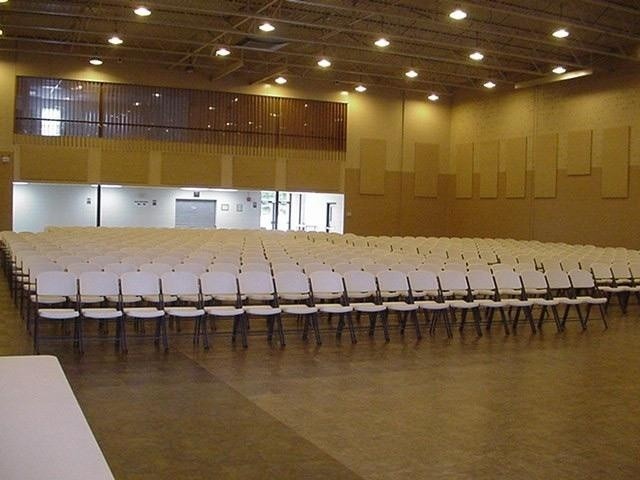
<box><xmin>86</xmin><ymin>0</ymin><xmax>570</xmax><ymax>102</ymax></box>
